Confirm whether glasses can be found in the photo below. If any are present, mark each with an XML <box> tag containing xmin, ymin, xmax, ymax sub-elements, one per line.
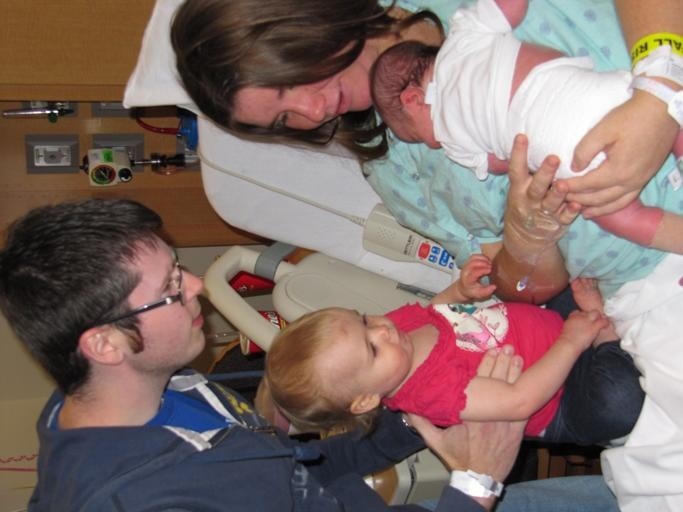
<box><xmin>99</xmin><ymin>247</ymin><xmax>188</xmax><ymax>322</ymax></box>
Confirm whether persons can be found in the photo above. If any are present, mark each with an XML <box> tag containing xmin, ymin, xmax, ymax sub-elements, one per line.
<box><xmin>365</xmin><ymin>0</ymin><xmax>683</xmax><ymax>259</ymax></box>
<box><xmin>0</xmin><ymin>194</ymin><xmax>620</xmax><ymax>511</ymax></box>
<box><xmin>166</xmin><ymin>0</ymin><xmax>682</xmax><ymax>308</ymax></box>
<box><xmin>264</xmin><ymin>254</ymin><xmax>644</xmax><ymax>450</ymax></box>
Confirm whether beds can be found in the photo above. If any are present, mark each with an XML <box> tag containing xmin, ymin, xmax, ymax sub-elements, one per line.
<box><xmin>114</xmin><ymin>1</ymin><xmax>682</xmax><ymax>445</ymax></box>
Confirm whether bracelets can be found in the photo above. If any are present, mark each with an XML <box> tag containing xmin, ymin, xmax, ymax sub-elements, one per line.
<box><xmin>446</xmin><ymin>469</ymin><xmax>507</xmax><ymax>499</ymax></box>
<box><xmin>626</xmin><ymin>31</ymin><xmax>682</xmax><ymax>132</ymax></box>
